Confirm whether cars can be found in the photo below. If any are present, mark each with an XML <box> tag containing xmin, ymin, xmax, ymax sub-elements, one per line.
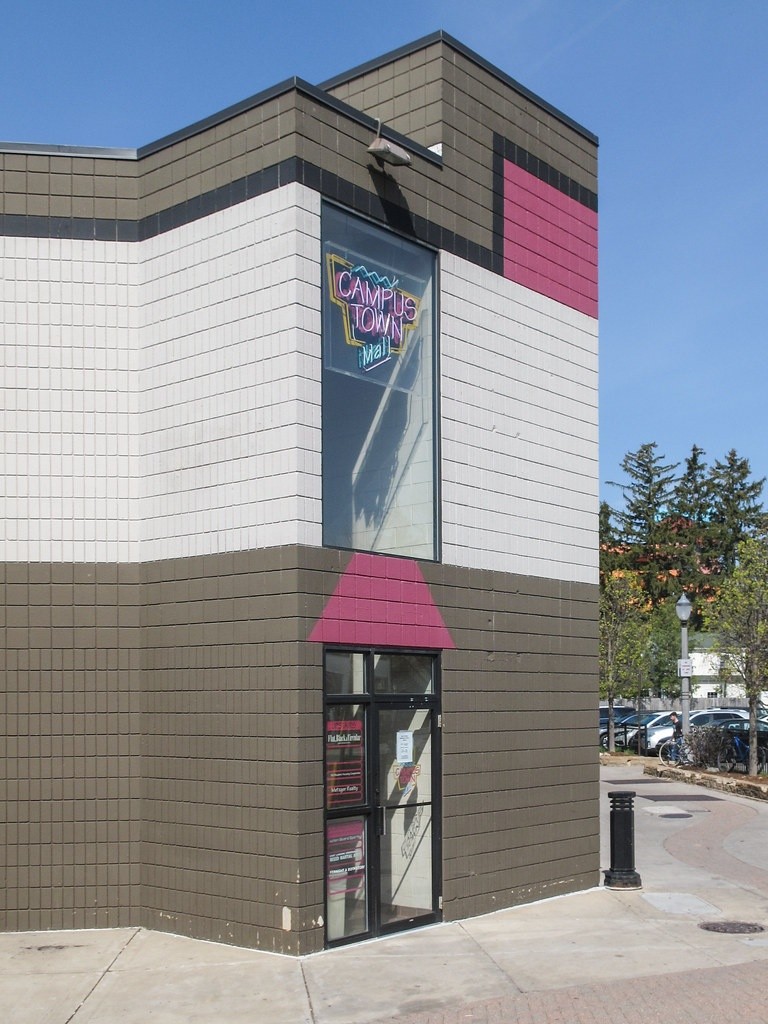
<box><xmin>598</xmin><ymin>706</ymin><xmax>687</xmax><ymax>751</ymax></box>
<box><xmin>637</xmin><ymin>709</ymin><xmax>754</xmax><ymax>758</ymax></box>
<box><xmin>701</xmin><ymin>719</ymin><xmax>768</xmax><ymax>762</ymax></box>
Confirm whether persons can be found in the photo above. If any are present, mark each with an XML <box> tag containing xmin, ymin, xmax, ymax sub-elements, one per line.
<box><xmin>669</xmin><ymin>712</ymin><xmax>682</xmax><ymax>760</ymax></box>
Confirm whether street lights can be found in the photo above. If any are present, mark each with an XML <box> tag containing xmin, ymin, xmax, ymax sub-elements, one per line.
<box><xmin>674</xmin><ymin>592</ymin><xmax>693</xmax><ymax>755</ymax></box>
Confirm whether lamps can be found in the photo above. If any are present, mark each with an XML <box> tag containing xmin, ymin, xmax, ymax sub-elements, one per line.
<box><xmin>366</xmin><ymin>118</ymin><xmax>412</xmax><ymax>168</ymax></box>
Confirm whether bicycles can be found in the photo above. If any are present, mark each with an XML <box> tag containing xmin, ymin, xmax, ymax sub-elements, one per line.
<box><xmin>659</xmin><ymin>726</ymin><xmax>707</xmax><ymax>768</ymax></box>
<box><xmin>714</xmin><ymin>729</ymin><xmax>766</xmax><ymax>775</ymax></box>
